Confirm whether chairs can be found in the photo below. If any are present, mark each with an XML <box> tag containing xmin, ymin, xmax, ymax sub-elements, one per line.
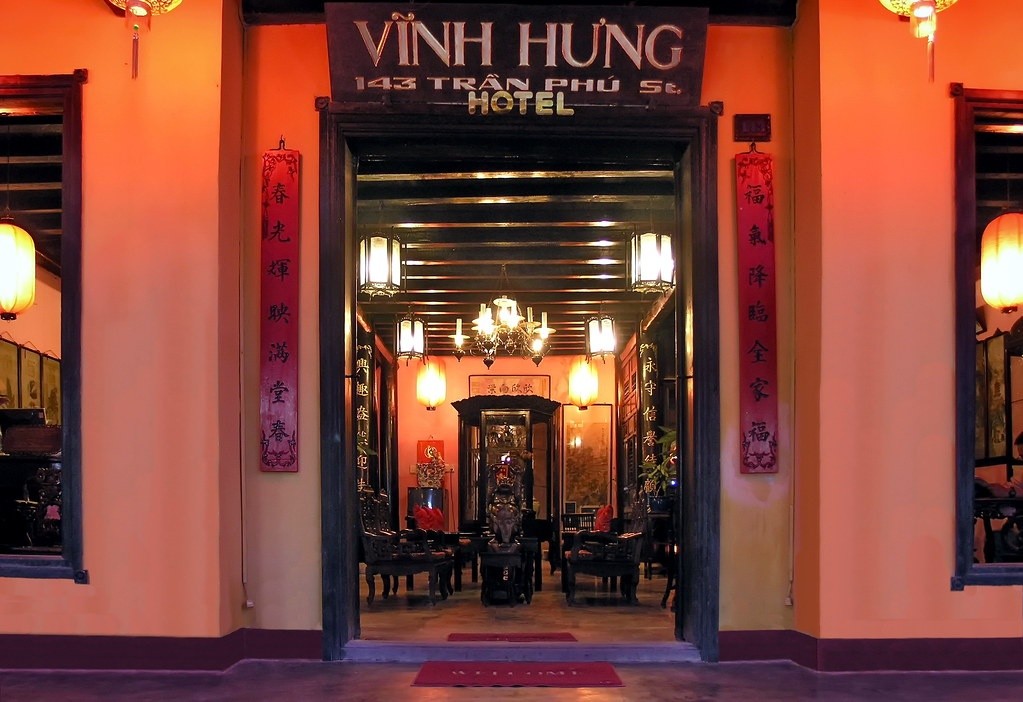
<box><xmin>565</xmin><ymin>484</ymin><xmax>649</xmax><ymax>606</ymax></box>
<box><xmin>356</xmin><ymin>484</ymin><xmax>450</xmax><ymax>605</ymax></box>
<box><xmin>377</xmin><ymin>488</ymin><xmax>463</xmax><ymax>596</ymax></box>
<box><xmin>561</xmin><ymin>490</ymin><xmax>641</xmax><ymax>593</ymax></box>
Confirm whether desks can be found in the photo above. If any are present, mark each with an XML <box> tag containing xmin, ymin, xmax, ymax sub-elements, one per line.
<box><xmin>643</xmin><ymin>512</ymin><xmax>674</xmax><ymax>579</ymax></box>
<box><xmin>974</xmin><ymin>464</ymin><xmax>1023</xmax><ymax>563</ymax></box>
<box><xmin>471</xmin><ymin>537</ymin><xmax>542</xmax><ymax>591</ymax></box>
<box><xmin>560</xmin><ymin>513</ymin><xmax>594</xmax><ymax>565</ymax></box>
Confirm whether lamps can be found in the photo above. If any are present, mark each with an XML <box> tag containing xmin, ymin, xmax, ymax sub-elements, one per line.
<box><xmin>391</xmin><ymin>305</ymin><xmax>426</xmax><ymax>367</ymax></box>
<box><xmin>622</xmin><ymin>200</ymin><xmax>676</xmax><ymax>301</ymax></box>
<box><xmin>583</xmin><ymin>298</ymin><xmax>618</xmax><ymax>364</ymax></box>
<box><xmin>447</xmin><ymin>263</ymin><xmax>556</xmax><ymax>370</ymax></box>
<box><xmin>357</xmin><ymin>200</ymin><xmax>403</xmax><ymax>302</ymax></box>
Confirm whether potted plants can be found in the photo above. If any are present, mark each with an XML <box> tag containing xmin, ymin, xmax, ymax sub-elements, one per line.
<box><xmin>638</xmin><ymin>451</ymin><xmax>677</xmax><ymax>514</ymax></box>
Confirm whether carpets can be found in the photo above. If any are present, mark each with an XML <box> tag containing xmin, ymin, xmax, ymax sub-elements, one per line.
<box><xmin>409</xmin><ymin>660</ymin><xmax>627</xmax><ymax>686</ymax></box>
<box><xmin>447</xmin><ymin>631</ymin><xmax>578</xmax><ymax>642</ymax></box>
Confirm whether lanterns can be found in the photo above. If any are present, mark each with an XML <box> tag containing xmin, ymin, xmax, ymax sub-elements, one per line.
<box><xmin>879</xmin><ymin>0</ymin><xmax>957</xmax><ymax>82</ymax></box>
<box><xmin>569</xmin><ymin>355</ymin><xmax>599</xmax><ymax>410</ymax></box>
<box><xmin>981</xmin><ymin>206</ymin><xmax>1023</xmax><ymax>313</ymax></box>
<box><xmin>0</xmin><ymin>217</ymin><xmax>35</xmax><ymax>320</ymax></box>
<box><xmin>358</xmin><ymin>226</ymin><xmax>407</xmax><ymax>301</ymax></box>
<box><xmin>417</xmin><ymin>355</ymin><xmax>446</xmax><ymax>411</ymax></box>
<box><xmin>625</xmin><ymin>230</ymin><xmax>676</xmax><ymax>300</ymax></box>
<box><xmin>395</xmin><ymin>313</ymin><xmax>429</xmax><ymax>368</ymax></box>
<box><xmin>583</xmin><ymin>312</ymin><xmax>616</xmax><ymax>365</ymax></box>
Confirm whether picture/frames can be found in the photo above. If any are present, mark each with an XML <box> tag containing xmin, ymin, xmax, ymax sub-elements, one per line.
<box><xmin>42</xmin><ymin>353</ymin><xmax>62</xmax><ymax>428</ymax></box>
<box><xmin>975</xmin><ymin>337</ymin><xmax>988</xmax><ymax>468</ymax></box>
<box><xmin>19</xmin><ymin>344</ymin><xmax>43</xmax><ymax>409</ymax></box>
<box><xmin>984</xmin><ymin>326</ymin><xmax>1012</xmax><ymax>466</ymax></box>
<box><xmin>0</xmin><ymin>337</ymin><xmax>20</xmax><ymax>409</ymax></box>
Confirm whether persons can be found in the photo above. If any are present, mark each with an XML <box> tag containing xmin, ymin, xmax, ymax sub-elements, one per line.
<box><xmin>1008</xmin><ymin>431</ymin><xmax>1023</xmax><ymax>494</ymax></box>
